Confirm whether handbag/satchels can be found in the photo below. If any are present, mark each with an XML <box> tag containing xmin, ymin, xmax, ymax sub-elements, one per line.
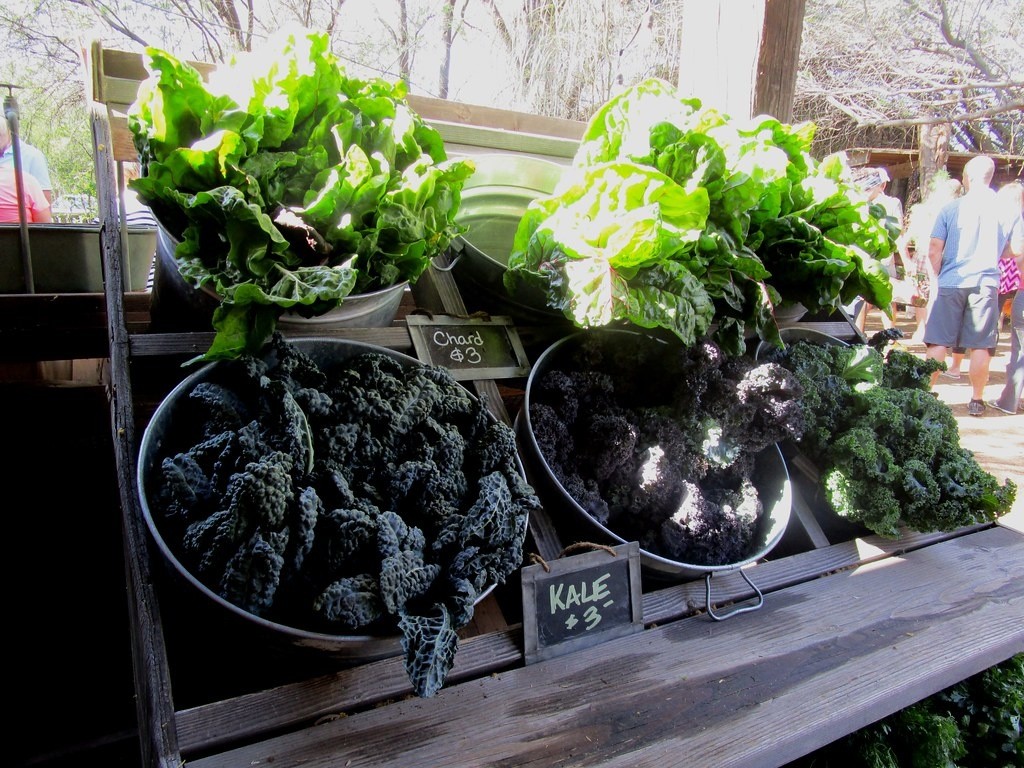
<box><xmin>996</xmin><ymin>257</ymin><xmax>1021</xmax><ymax>294</ymax></box>
<box><xmin>887</xmin><ymin>255</ymin><xmax>930</xmax><ymax>308</ymax></box>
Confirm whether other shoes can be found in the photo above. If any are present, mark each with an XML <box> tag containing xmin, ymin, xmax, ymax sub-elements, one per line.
<box><xmin>987</xmin><ymin>398</ymin><xmax>1016</xmax><ymax>415</ymax></box>
<box><xmin>905</xmin><ymin>311</ymin><xmax>916</xmax><ymax>319</ymax></box>
<box><xmin>967</xmin><ymin>401</ymin><xmax>986</xmax><ymax>415</ymax></box>
<box><xmin>1018</xmin><ymin>400</ymin><xmax>1024</xmax><ymax>410</ymax></box>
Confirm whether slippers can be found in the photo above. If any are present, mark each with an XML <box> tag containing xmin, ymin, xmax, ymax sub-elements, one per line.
<box><xmin>941</xmin><ymin>368</ymin><xmax>960</xmax><ymax>379</ymax></box>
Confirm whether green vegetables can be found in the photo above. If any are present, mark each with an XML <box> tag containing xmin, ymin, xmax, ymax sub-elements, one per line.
<box><xmin>115</xmin><ymin>23</ymin><xmax>1017</xmax><ymax>563</ymax></box>
<box><xmin>765</xmin><ymin>651</ymin><xmax>1024</xmax><ymax>768</ymax></box>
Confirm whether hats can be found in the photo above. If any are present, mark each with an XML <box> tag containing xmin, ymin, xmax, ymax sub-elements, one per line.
<box><xmin>877</xmin><ymin>167</ymin><xmax>890</xmax><ymax>183</ymax></box>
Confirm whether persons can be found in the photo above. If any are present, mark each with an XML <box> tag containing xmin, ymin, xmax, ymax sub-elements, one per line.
<box><xmin>91</xmin><ymin>160</ymin><xmax>158</xmax><ymax>293</ymax></box>
<box><xmin>0</xmin><ymin>114</ymin><xmax>53</xmax><ymax>222</ymax></box>
<box><xmin>842</xmin><ymin>156</ymin><xmax>1024</xmax><ymax>416</ymax></box>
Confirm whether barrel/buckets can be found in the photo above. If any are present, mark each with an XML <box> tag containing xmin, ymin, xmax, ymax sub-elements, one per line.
<box><xmin>149</xmin><ymin>197</ymin><xmax>411</xmax><ymax>332</ymax></box>
<box><xmin>431</xmin><ymin>183</ymin><xmax>570</xmax><ymax>322</ymax></box>
<box><xmin>516</xmin><ymin>329</ymin><xmax>794</xmax><ymax>620</ymax></box>
<box><xmin>755</xmin><ymin>327</ymin><xmax>850</xmax><ymax>485</ymax></box>
<box><xmin>135</xmin><ymin>338</ymin><xmax>528</xmax><ymax>666</ymax></box>
